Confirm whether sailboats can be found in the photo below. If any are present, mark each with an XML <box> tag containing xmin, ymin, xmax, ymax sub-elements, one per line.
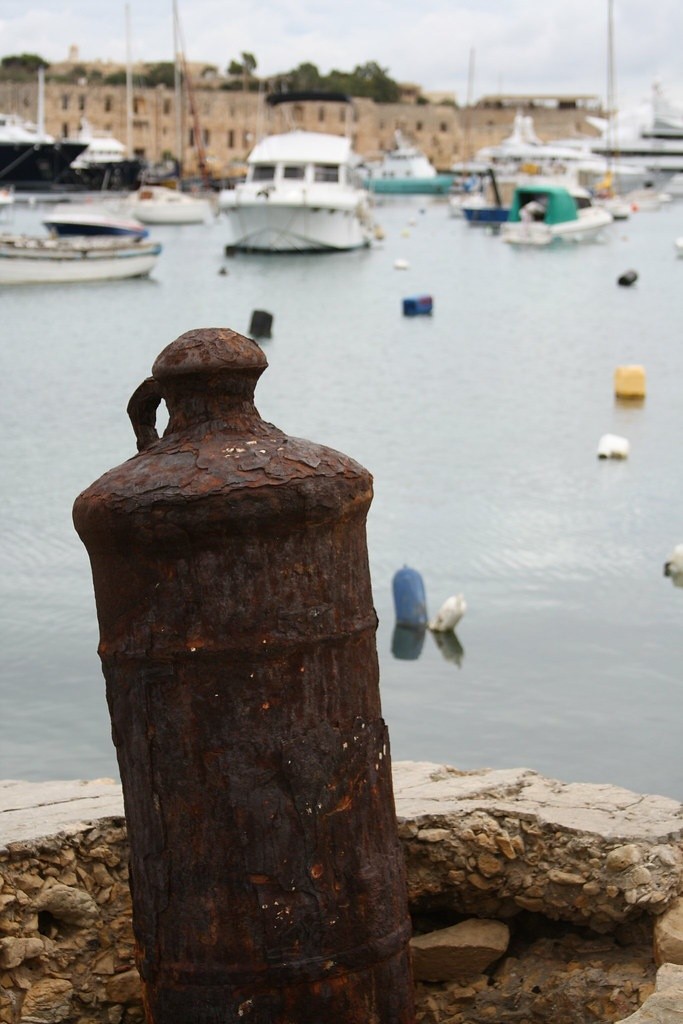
<box><xmin>121</xmin><ymin>1</ymin><xmax>245</xmax><ymax>192</ymax></box>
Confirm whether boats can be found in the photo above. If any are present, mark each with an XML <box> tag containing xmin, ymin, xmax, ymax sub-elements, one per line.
<box><xmin>368</xmin><ymin>130</ymin><xmax>454</xmax><ymax>196</ymax></box>
<box><xmin>0</xmin><ymin>65</ymin><xmax>168</xmax><ymax>284</ymax></box>
<box><xmin>453</xmin><ymin>1</ymin><xmax>683</xmax><ymax>245</ymax></box>
<box><xmin>128</xmin><ymin>181</ymin><xmax>213</xmax><ymax>225</ymax></box>
<box><xmin>215</xmin><ymin>91</ymin><xmax>378</xmax><ymax>255</ymax></box>
